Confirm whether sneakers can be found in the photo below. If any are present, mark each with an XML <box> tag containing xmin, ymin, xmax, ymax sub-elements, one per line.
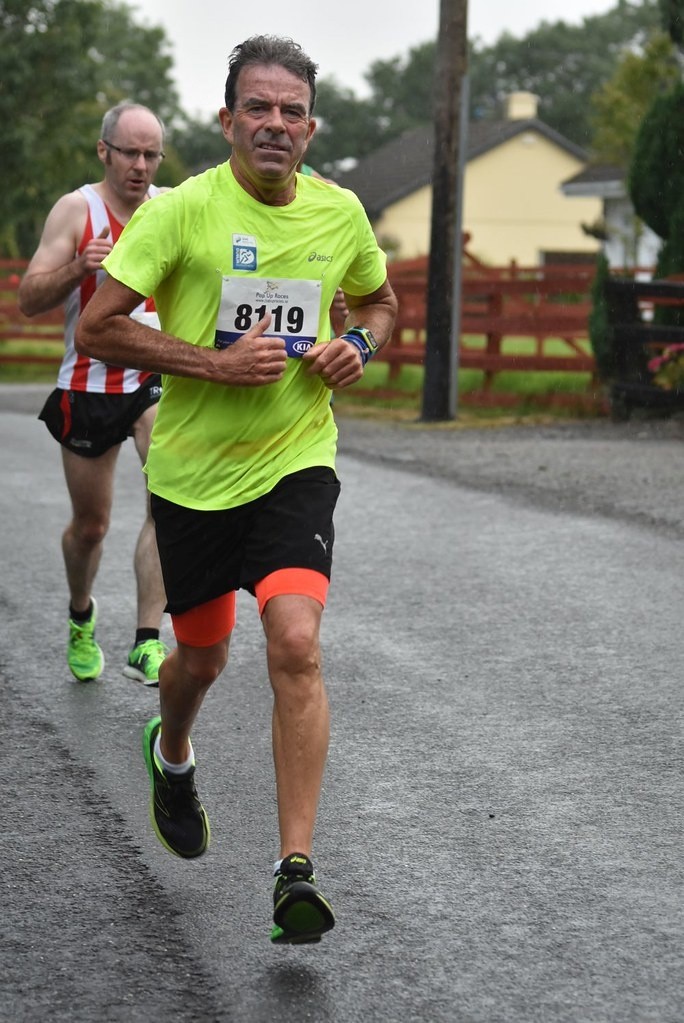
<box><xmin>120</xmin><ymin>638</ymin><xmax>168</xmax><ymax>684</ymax></box>
<box><xmin>270</xmin><ymin>853</ymin><xmax>334</xmax><ymax>946</ymax></box>
<box><xmin>144</xmin><ymin>717</ymin><xmax>208</xmax><ymax>861</ymax></box>
<box><xmin>69</xmin><ymin>596</ymin><xmax>105</xmax><ymax>682</ymax></box>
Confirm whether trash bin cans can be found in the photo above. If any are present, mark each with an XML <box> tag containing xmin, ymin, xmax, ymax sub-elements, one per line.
<box><xmin>601</xmin><ymin>275</ymin><xmax>684</xmax><ymax>423</ymax></box>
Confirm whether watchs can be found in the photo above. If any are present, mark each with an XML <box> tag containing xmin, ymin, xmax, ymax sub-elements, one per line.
<box><xmin>344</xmin><ymin>325</ymin><xmax>380</xmax><ymax>360</ymax></box>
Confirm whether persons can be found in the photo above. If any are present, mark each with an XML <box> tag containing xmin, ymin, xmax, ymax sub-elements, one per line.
<box><xmin>16</xmin><ymin>102</ymin><xmax>175</xmax><ymax>690</ymax></box>
<box><xmin>71</xmin><ymin>33</ymin><xmax>400</xmax><ymax>946</ymax></box>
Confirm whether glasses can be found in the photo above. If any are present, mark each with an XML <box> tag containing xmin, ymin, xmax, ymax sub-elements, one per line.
<box><xmin>103</xmin><ymin>141</ymin><xmax>165</xmax><ymax>163</ymax></box>
